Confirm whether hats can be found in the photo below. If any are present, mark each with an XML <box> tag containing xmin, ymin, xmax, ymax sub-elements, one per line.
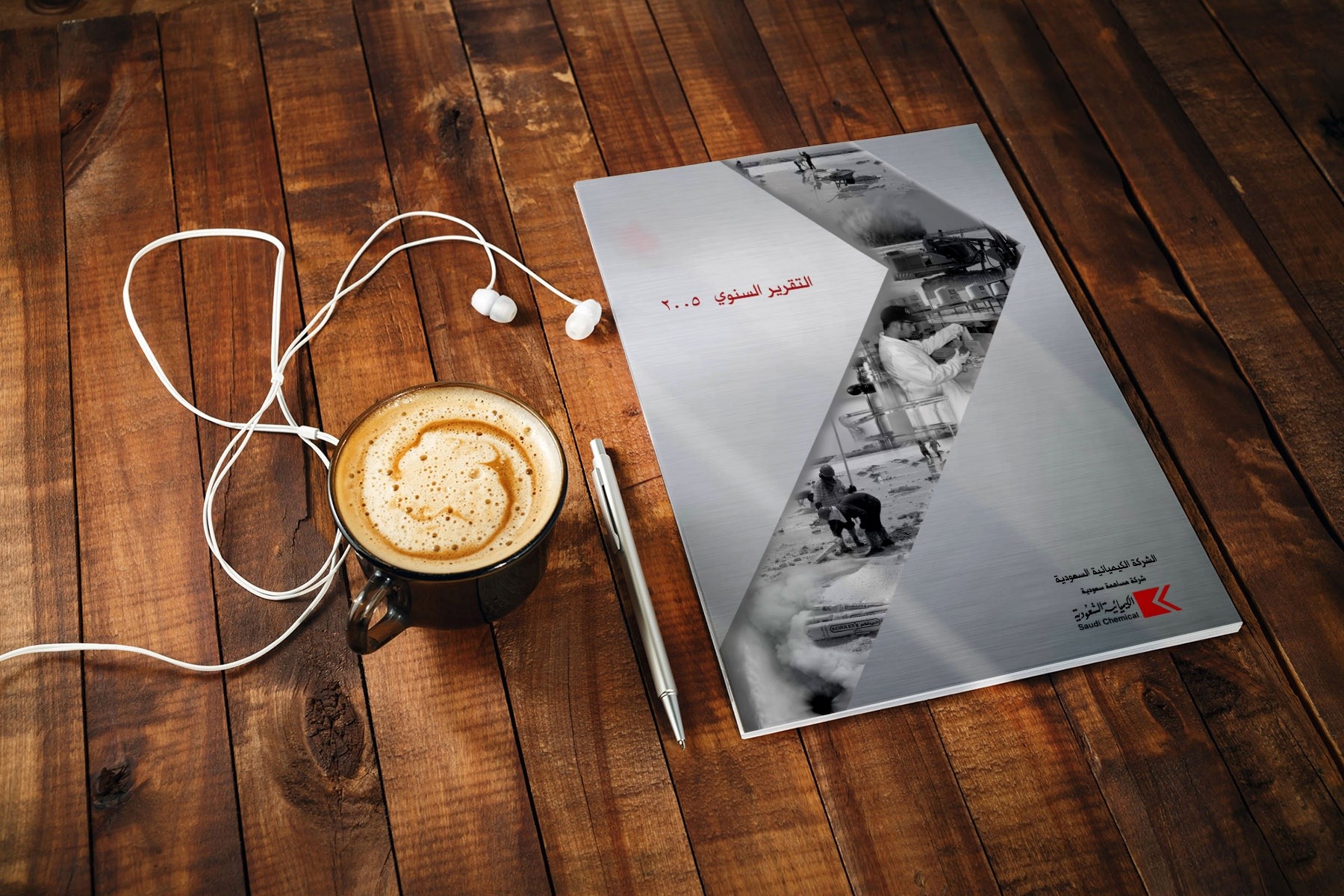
<box><xmin>880</xmin><ymin>306</ymin><xmax>927</xmax><ymax>322</ymax></box>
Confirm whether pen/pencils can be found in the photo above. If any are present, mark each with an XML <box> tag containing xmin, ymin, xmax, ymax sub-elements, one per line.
<box><xmin>589</xmin><ymin>439</ymin><xmax>686</xmax><ymax>750</ymax></box>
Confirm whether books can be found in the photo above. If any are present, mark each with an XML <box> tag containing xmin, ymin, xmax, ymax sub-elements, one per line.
<box><xmin>574</xmin><ymin>122</ymin><xmax>1242</xmax><ymax>737</ymax></box>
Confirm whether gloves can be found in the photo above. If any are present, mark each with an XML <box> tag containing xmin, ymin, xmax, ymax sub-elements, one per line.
<box><xmin>951</xmin><ymin>323</ymin><xmax>963</xmax><ymax>336</ymax></box>
<box><xmin>848</xmin><ymin>485</ymin><xmax>856</xmax><ymax>493</ymax></box>
<box><xmin>953</xmin><ymin>350</ymin><xmax>971</xmax><ymax>365</ymax></box>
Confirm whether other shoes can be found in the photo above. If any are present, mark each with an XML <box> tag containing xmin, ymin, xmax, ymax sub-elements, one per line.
<box><xmin>866</xmin><ymin>547</ymin><xmax>884</xmax><ymax>557</ymax></box>
<box><xmin>842</xmin><ymin>546</ymin><xmax>851</xmax><ymax>553</ymax></box>
<box><xmin>856</xmin><ymin>542</ymin><xmax>865</xmax><ymax>547</ymax></box>
<box><xmin>881</xmin><ymin>539</ymin><xmax>894</xmax><ymax>546</ymax></box>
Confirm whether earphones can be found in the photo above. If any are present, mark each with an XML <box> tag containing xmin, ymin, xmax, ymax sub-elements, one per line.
<box><xmin>565</xmin><ymin>298</ymin><xmax>602</xmax><ymax>340</ymax></box>
<box><xmin>471</xmin><ymin>289</ymin><xmax>518</xmax><ymax>323</ymax></box>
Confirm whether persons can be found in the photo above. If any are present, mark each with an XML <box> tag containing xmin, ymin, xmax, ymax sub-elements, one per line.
<box><xmin>918</xmin><ymin>436</ymin><xmax>940</xmax><ymax>459</ymax></box>
<box><xmin>794</xmin><ymin>152</ymin><xmax>816</xmax><ymax>170</ymax></box>
<box><xmin>879</xmin><ymin>305</ymin><xmax>970</xmax><ymax>440</ymax></box>
<box><xmin>815</xmin><ymin>465</ymin><xmax>894</xmax><ymax>557</ymax></box>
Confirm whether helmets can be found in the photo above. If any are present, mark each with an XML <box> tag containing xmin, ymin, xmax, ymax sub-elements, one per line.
<box><xmin>817</xmin><ymin>507</ymin><xmax>830</xmax><ymax>519</ymax></box>
<box><xmin>820</xmin><ymin>464</ymin><xmax>834</xmax><ymax>477</ymax></box>
